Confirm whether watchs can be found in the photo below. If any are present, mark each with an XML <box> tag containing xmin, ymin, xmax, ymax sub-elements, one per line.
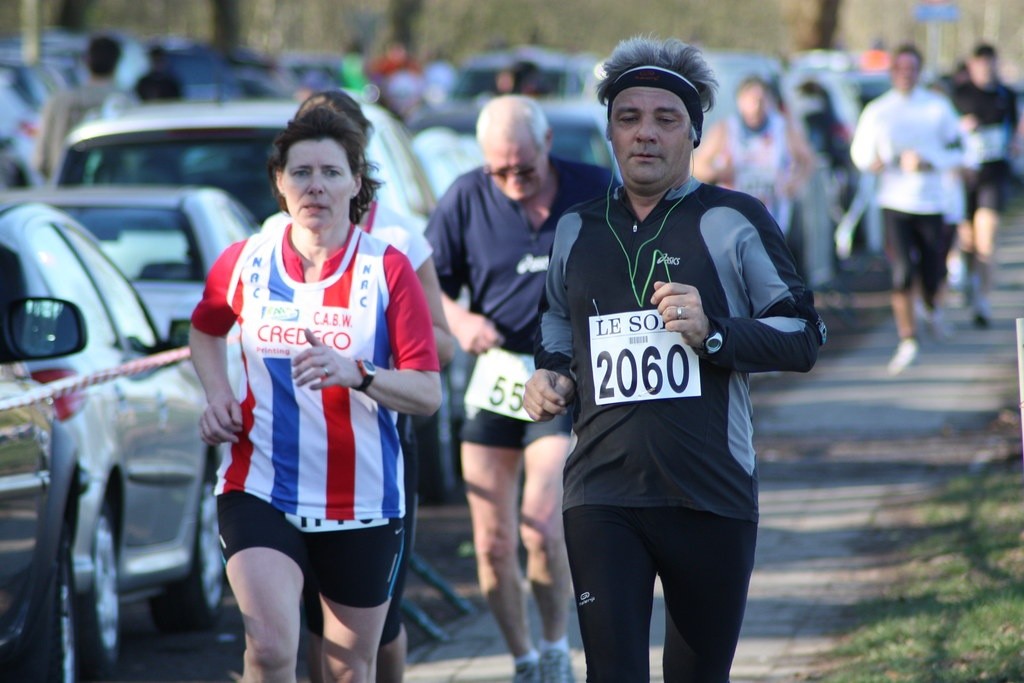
<box><xmin>693</xmin><ymin>319</ymin><xmax>722</xmax><ymax>354</ymax></box>
<box><xmin>351</xmin><ymin>359</ymin><xmax>376</xmax><ymax>392</ymax></box>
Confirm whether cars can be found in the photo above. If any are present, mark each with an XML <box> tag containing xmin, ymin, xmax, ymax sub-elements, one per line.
<box><xmin>0</xmin><ymin>184</ymin><xmax>266</xmax><ymax>349</ymax></box>
<box><xmin>0</xmin><ymin>27</ymin><xmax>960</xmax><ymax>512</ymax></box>
<box><xmin>0</xmin><ymin>198</ymin><xmax>228</xmax><ymax>683</ymax></box>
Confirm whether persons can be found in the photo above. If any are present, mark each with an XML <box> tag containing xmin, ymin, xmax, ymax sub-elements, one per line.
<box><xmin>339</xmin><ymin>39</ymin><xmax>558</xmax><ymax>132</ymax></box>
<box><xmin>423</xmin><ymin>93</ymin><xmax>622</xmax><ymax>683</ymax></box>
<box><xmin>690</xmin><ymin>73</ymin><xmax>861</xmax><ymax>315</ymax></box>
<box><xmin>31</xmin><ymin>35</ymin><xmax>141</xmax><ymax>181</ymax></box>
<box><xmin>133</xmin><ymin>44</ymin><xmax>187</xmax><ymax>100</ymax></box>
<box><xmin>518</xmin><ymin>35</ymin><xmax>831</xmax><ymax>683</ymax></box>
<box><xmin>185</xmin><ymin>91</ymin><xmax>452</xmax><ymax>683</ymax></box>
<box><xmin>850</xmin><ymin>43</ymin><xmax>1024</xmax><ymax>375</ymax></box>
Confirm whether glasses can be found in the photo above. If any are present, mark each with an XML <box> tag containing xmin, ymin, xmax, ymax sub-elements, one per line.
<box><xmin>482</xmin><ymin>137</ymin><xmax>547</xmax><ymax>177</ymax></box>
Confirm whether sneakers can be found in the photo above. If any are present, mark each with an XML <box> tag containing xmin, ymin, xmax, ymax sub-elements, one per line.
<box><xmin>541</xmin><ymin>650</ymin><xmax>573</xmax><ymax>683</ymax></box>
<box><xmin>515</xmin><ymin>658</ymin><xmax>542</xmax><ymax>683</ymax></box>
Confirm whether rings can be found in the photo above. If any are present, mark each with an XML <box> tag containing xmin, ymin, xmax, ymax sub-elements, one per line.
<box><xmin>323</xmin><ymin>367</ymin><xmax>329</xmax><ymax>375</ymax></box>
<box><xmin>677</xmin><ymin>306</ymin><xmax>682</xmax><ymax>318</ymax></box>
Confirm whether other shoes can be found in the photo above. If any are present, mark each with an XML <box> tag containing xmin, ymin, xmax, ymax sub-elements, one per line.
<box><xmin>887</xmin><ymin>339</ymin><xmax>917</xmax><ymax>377</ymax></box>
